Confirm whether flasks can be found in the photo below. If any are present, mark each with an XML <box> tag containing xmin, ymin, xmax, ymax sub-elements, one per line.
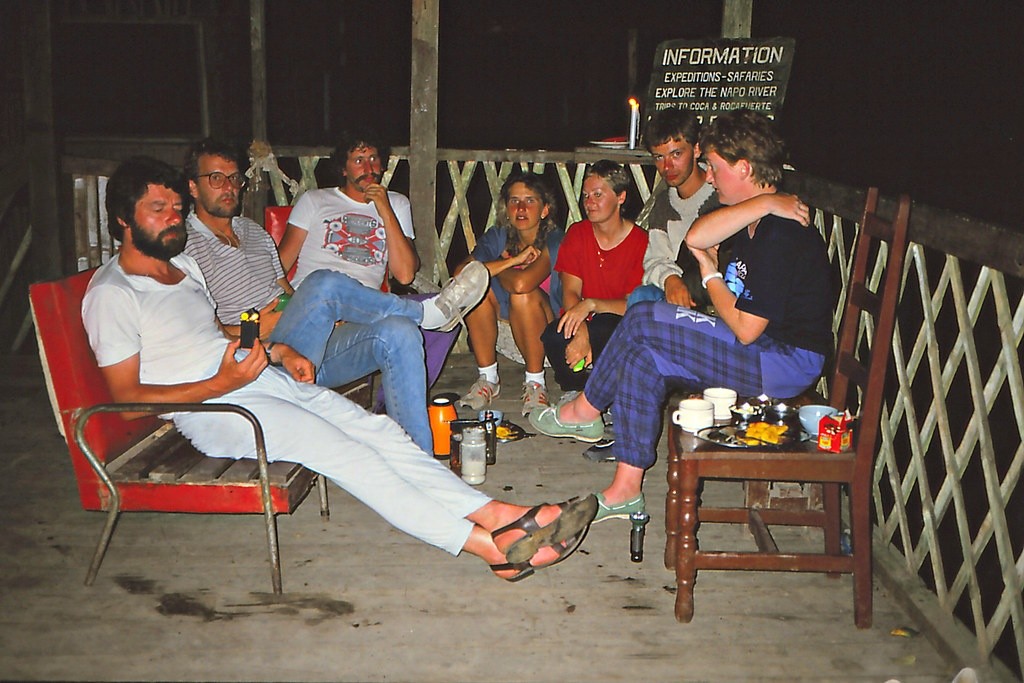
<box><xmin>428</xmin><ymin>393</ymin><xmax>461</xmax><ymax>460</ymax></box>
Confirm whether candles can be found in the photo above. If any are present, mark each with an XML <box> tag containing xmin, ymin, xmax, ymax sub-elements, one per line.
<box><xmin>629</xmin><ymin>99</ymin><xmax>638</xmax><ymax>150</ymax></box>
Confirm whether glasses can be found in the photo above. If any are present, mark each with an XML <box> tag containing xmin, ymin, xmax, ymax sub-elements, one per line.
<box><xmin>194</xmin><ymin>171</ymin><xmax>246</xmax><ymax>190</ymax></box>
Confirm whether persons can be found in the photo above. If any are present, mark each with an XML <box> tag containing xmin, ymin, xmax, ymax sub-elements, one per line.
<box><xmin>277</xmin><ymin>134</ymin><xmax>462</xmax><ymax>416</ymax></box>
<box><xmin>175</xmin><ymin>137</ymin><xmax>491</xmax><ymax>456</ymax></box>
<box><xmin>581</xmin><ymin>106</ymin><xmax>732</xmax><ymax>465</ymax></box>
<box><xmin>529</xmin><ymin>108</ymin><xmax>836</xmax><ymax>524</ymax></box>
<box><xmin>79</xmin><ymin>156</ymin><xmax>599</xmax><ymax>582</ymax></box>
<box><xmin>540</xmin><ymin>158</ymin><xmax>661</xmax><ymax>429</ymax></box>
<box><xmin>454</xmin><ymin>172</ymin><xmax>569</xmax><ymax>415</ymax></box>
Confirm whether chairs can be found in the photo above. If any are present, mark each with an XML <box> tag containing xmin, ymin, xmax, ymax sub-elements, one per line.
<box><xmin>264</xmin><ymin>203</ymin><xmax>438</xmax><ymax>417</ymax></box>
<box><xmin>31</xmin><ymin>262</ymin><xmax>333</xmax><ymax>596</ymax></box>
<box><xmin>664</xmin><ymin>183</ymin><xmax>913</xmax><ymax>631</ymax></box>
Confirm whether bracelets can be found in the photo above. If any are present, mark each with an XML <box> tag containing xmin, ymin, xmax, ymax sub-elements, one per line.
<box><xmin>702</xmin><ymin>272</ymin><xmax>723</xmax><ymax>288</ymax></box>
<box><xmin>265</xmin><ymin>341</ymin><xmax>285</xmax><ymax>366</ymax></box>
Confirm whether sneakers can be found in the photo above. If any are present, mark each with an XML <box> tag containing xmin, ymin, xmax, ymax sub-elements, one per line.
<box><xmin>459</xmin><ymin>373</ymin><xmax>501</xmax><ymax>411</ymax></box>
<box><xmin>520</xmin><ymin>380</ymin><xmax>550</xmax><ymax>418</ymax></box>
<box><xmin>420</xmin><ymin>260</ymin><xmax>490</xmax><ymax>333</ymax></box>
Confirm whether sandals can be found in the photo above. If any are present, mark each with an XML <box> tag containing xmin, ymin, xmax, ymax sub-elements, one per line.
<box><xmin>491</xmin><ymin>493</ymin><xmax>599</xmax><ymax>565</ymax></box>
<box><xmin>489</xmin><ymin>524</ymin><xmax>589</xmax><ymax>583</ymax></box>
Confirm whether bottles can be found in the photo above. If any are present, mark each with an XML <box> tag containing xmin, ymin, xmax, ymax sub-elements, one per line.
<box><xmin>484</xmin><ymin>411</ymin><xmax>496</xmax><ymax>465</ymax></box>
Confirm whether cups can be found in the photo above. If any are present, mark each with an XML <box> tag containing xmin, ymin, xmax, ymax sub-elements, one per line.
<box><xmin>459</xmin><ymin>426</ymin><xmax>487</xmax><ymax>485</ymax></box>
<box><xmin>672</xmin><ymin>399</ymin><xmax>714</xmax><ymax>433</ymax></box>
<box><xmin>704</xmin><ymin>384</ymin><xmax>736</xmax><ymax>421</ymax></box>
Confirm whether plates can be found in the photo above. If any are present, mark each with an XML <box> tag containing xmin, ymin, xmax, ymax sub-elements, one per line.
<box><xmin>693</xmin><ymin>425</ymin><xmax>810</xmax><ymax>449</ymax></box>
<box><xmin>588</xmin><ymin>140</ymin><xmax>630</xmax><ymax>150</ymax></box>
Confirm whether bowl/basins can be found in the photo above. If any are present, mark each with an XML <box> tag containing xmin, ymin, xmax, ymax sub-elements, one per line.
<box><xmin>729</xmin><ymin>401</ymin><xmax>797</xmax><ymax>425</ymax></box>
<box><xmin>798</xmin><ymin>406</ymin><xmax>838</xmax><ymax>436</ymax></box>
<box><xmin>478</xmin><ymin>411</ymin><xmax>503</xmax><ymax>426</ymax></box>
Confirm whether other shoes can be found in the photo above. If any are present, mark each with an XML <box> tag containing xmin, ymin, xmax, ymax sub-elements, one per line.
<box><xmin>528</xmin><ymin>399</ymin><xmax>604</xmax><ymax>443</ymax></box>
<box><xmin>588</xmin><ymin>491</ymin><xmax>646</xmax><ymax>525</ymax></box>
